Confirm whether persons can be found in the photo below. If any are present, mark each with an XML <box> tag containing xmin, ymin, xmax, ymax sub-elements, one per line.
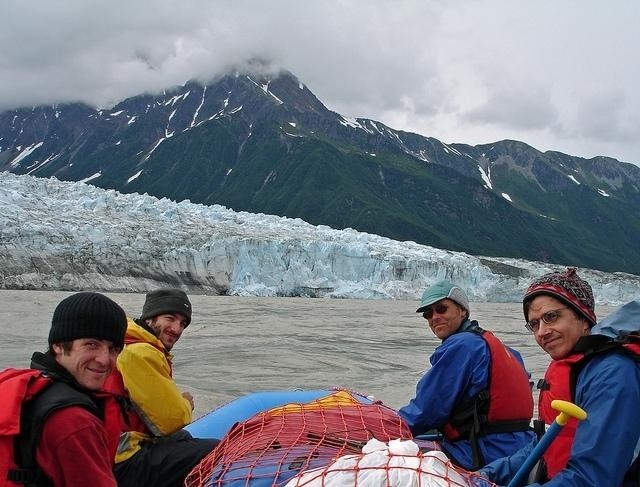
<box><xmin>395</xmin><ymin>279</ymin><xmax>536</xmax><ymax>470</ymax></box>
<box><xmin>112</xmin><ymin>287</ymin><xmax>223</xmax><ymax>487</ymax></box>
<box><xmin>0</xmin><ymin>291</ymin><xmax>128</xmax><ymax>487</ymax></box>
<box><xmin>465</xmin><ymin>268</ymin><xmax>640</xmax><ymax>487</ymax></box>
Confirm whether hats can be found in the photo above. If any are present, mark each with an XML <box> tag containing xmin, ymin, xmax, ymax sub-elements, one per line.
<box><xmin>140</xmin><ymin>287</ymin><xmax>193</xmax><ymax>326</ymax></box>
<box><xmin>46</xmin><ymin>292</ymin><xmax>127</xmax><ymax>352</ymax></box>
<box><xmin>414</xmin><ymin>281</ymin><xmax>471</xmax><ymax>318</ymax></box>
<box><xmin>522</xmin><ymin>267</ymin><xmax>597</xmax><ymax>328</ymax></box>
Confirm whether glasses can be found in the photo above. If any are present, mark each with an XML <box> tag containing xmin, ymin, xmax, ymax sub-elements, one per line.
<box><xmin>423</xmin><ymin>301</ymin><xmax>455</xmax><ymax>320</ymax></box>
<box><xmin>525</xmin><ymin>306</ymin><xmax>568</xmax><ymax>333</ymax></box>
